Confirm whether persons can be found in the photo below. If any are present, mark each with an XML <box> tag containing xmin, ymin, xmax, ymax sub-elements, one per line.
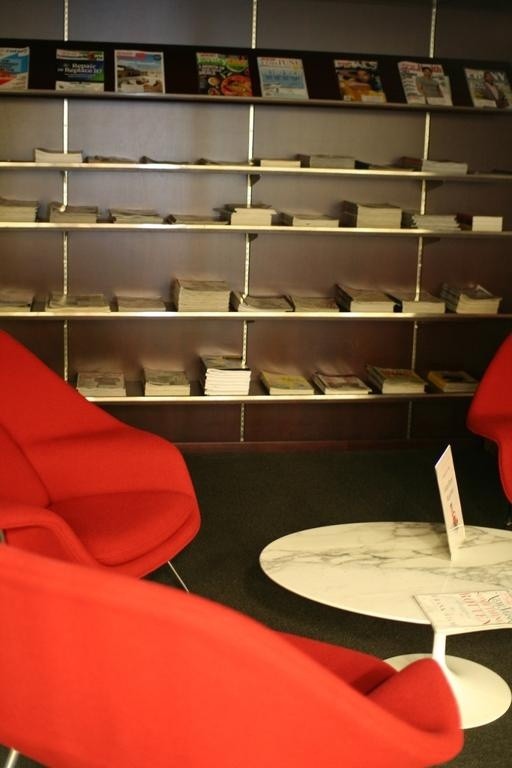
<box><xmin>354</xmin><ymin>66</ymin><xmax>373</xmax><ymax>90</ymax></box>
<box><xmin>484</xmin><ymin>71</ymin><xmax>509</xmax><ymax>109</ymax></box>
<box><xmin>416</xmin><ymin>66</ymin><xmax>443</xmax><ymax>98</ymax></box>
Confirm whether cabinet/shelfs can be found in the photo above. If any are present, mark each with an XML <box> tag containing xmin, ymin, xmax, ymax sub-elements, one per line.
<box><xmin>1</xmin><ymin>40</ymin><xmax>510</xmax><ymax>455</ymax></box>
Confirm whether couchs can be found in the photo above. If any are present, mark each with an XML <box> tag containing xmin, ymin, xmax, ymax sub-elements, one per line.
<box><xmin>466</xmin><ymin>331</ymin><xmax>512</xmax><ymax>506</ymax></box>
<box><xmin>0</xmin><ymin>545</ymin><xmax>463</xmax><ymax>768</ymax></box>
<box><xmin>0</xmin><ymin>335</ymin><xmax>202</xmax><ymax>575</ymax></box>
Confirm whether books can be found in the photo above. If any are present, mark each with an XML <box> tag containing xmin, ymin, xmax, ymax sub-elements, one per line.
<box><xmin>428</xmin><ymin>370</ymin><xmax>480</xmax><ymax>393</ymax></box>
<box><xmin>256</xmin><ymin>158</ymin><xmax>301</xmax><ymax>167</ymax></box>
<box><xmin>174</xmin><ymin>278</ymin><xmax>232</xmax><ymax>311</ymax></box>
<box><xmin>412</xmin><ymin>591</ymin><xmax>512</xmax><ymax>636</ymax></box>
<box><xmin>465</xmin><ymin>68</ymin><xmax>512</xmax><ymax>110</ymax></box>
<box><xmin>44</xmin><ymin>293</ymin><xmax>111</xmax><ymax>313</ymax></box>
<box><xmin>287</xmin><ymin>294</ymin><xmax>340</xmax><ymax>312</ymax></box>
<box><xmin>196</xmin><ymin>52</ymin><xmax>253</xmax><ymax>96</ymax></box>
<box><xmin>462</xmin><ymin>211</ymin><xmax>503</xmax><ymax>232</ymax></box>
<box><xmin>401</xmin><ymin>212</ymin><xmax>461</xmax><ymax>231</ymax></box>
<box><xmin>56</xmin><ymin>49</ymin><xmax>105</xmax><ymax>92</ymax></box>
<box><xmin>0</xmin><ymin>287</ymin><xmax>35</xmax><ymax>312</ymax></box>
<box><xmin>140</xmin><ymin>367</ymin><xmax>190</xmax><ymax>396</ymax></box>
<box><xmin>34</xmin><ymin>147</ymin><xmax>84</xmax><ymax>163</ymax></box>
<box><xmin>386</xmin><ymin>292</ymin><xmax>445</xmax><ymax>314</ymax></box>
<box><xmin>279</xmin><ymin>211</ymin><xmax>338</xmax><ymax>227</ymax></box>
<box><xmin>50</xmin><ymin>202</ymin><xmax>97</xmax><ymax>223</ymax></box>
<box><xmin>199</xmin><ymin>354</ymin><xmax>251</xmax><ymax>395</ymax></box>
<box><xmin>335</xmin><ymin>282</ymin><xmax>396</xmax><ymax>313</ymax></box>
<box><xmin>212</xmin><ymin>203</ymin><xmax>277</xmax><ymax>226</ymax></box>
<box><xmin>296</xmin><ymin>153</ymin><xmax>360</xmax><ymax>168</ymax></box>
<box><xmin>368</xmin><ymin>367</ymin><xmax>428</xmax><ymax>394</ymax></box>
<box><xmin>257</xmin><ymin>56</ymin><xmax>309</xmax><ymax>99</ymax></box>
<box><xmin>439</xmin><ymin>284</ymin><xmax>502</xmax><ymax>314</ymax></box>
<box><xmin>231</xmin><ymin>291</ymin><xmax>294</xmax><ymax>312</ymax></box>
<box><xmin>0</xmin><ymin>48</ymin><xmax>29</xmax><ymax>90</ymax></box>
<box><xmin>113</xmin><ymin>289</ymin><xmax>166</xmax><ymax>311</ymax></box>
<box><xmin>139</xmin><ymin>156</ymin><xmax>192</xmax><ymax>164</ymax></box>
<box><xmin>355</xmin><ymin>160</ymin><xmax>413</xmax><ymax>171</ymax></box>
<box><xmin>334</xmin><ymin>59</ymin><xmax>387</xmax><ymax>103</ymax></box>
<box><xmin>197</xmin><ymin>158</ymin><xmax>247</xmax><ymax>165</ymax></box>
<box><xmin>434</xmin><ymin>445</ymin><xmax>466</xmax><ymax>561</ymax></box>
<box><xmin>165</xmin><ymin>214</ymin><xmax>229</xmax><ymax>225</ymax></box>
<box><xmin>311</xmin><ymin>372</ymin><xmax>372</xmax><ymax>395</ymax></box>
<box><xmin>109</xmin><ymin>208</ymin><xmax>164</xmax><ymax>224</ymax></box>
<box><xmin>259</xmin><ymin>370</ymin><xmax>314</xmax><ymax>394</ymax></box>
<box><xmin>398</xmin><ymin>61</ymin><xmax>453</xmax><ymax>106</ymax></box>
<box><xmin>399</xmin><ymin>156</ymin><xmax>468</xmax><ymax>173</ymax></box>
<box><xmin>341</xmin><ymin>200</ymin><xmax>401</xmax><ymax>229</ymax></box>
<box><xmin>115</xmin><ymin>50</ymin><xmax>165</xmax><ymax>94</ymax></box>
<box><xmin>0</xmin><ymin>199</ymin><xmax>39</xmax><ymax>222</ymax></box>
<box><xmin>76</xmin><ymin>370</ymin><xmax>126</xmax><ymax>397</ymax></box>
<box><xmin>88</xmin><ymin>156</ymin><xmax>137</xmax><ymax>164</ymax></box>
